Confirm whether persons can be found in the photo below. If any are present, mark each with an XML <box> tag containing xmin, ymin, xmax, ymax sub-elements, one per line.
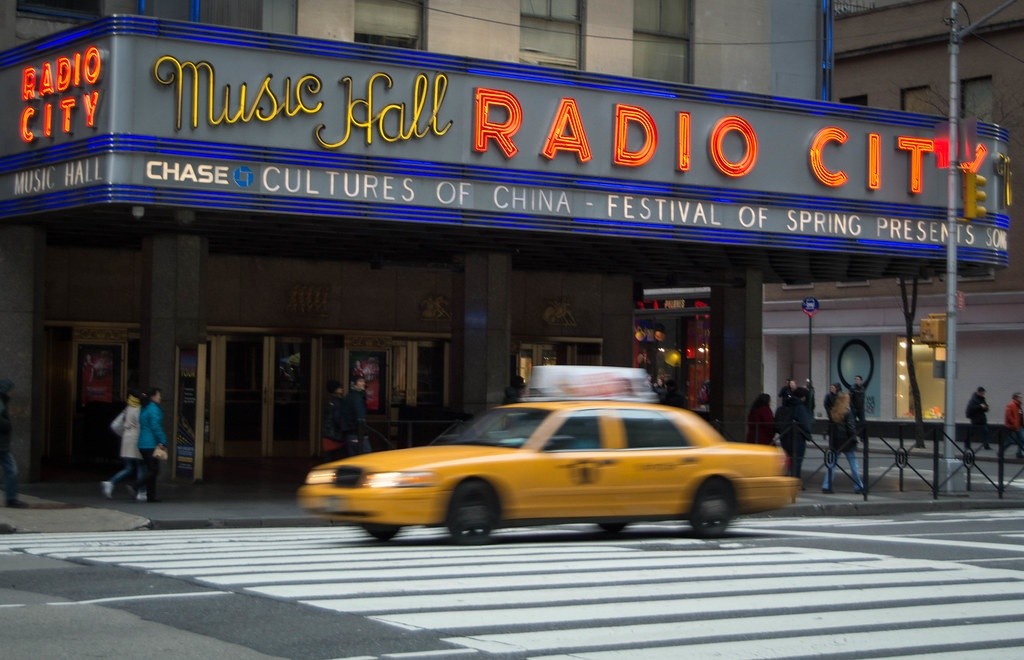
<box><xmin>775</xmin><ymin>386</ymin><xmax>814</xmax><ymax>491</ymax></box>
<box><xmin>99</xmin><ymin>385</ymin><xmax>150</xmax><ymax>499</ymax></box>
<box><xmin>823</xmin><ymin>382</ymin><xmax>842</xmax><ymax>420</ymax></box>
<box><xmin>997</xmin><ymin>392</ymin><xmax>1024</xmax><ymax>459</ymax></box>
<box><xmin>747</xmin><ymin>393</ymin><xmax>776</xmax><ymax>445</ymax></box>
<box><xmin>0</xmin><ymin>378</ymin><xmax>28</xmax><ymax>508</ymax></box>
<box><xmin>320</xmin><ymin>378</ymin><xmax>345</xmax><ymax>462</ymax></box>
<box><xmin>821</xmin><ymin>391</ymin><xmax>864</xmax><ymax>494</ymax></box>
<box><xmin>502</xmin><ymin>374</ymin><xmax>526</xmax><ymax>405</ymax></box>
<box><xmin>849</xmin><ymin>375</ymin><xmax>866</xmax><ymax>442</ymax></box>
<box><xmin>964</xmin><ymin>387</ymin><xmax>992</xmax><ymax>450</ymax></box>
<box><xmin>341</xmin><ymin>375</ymin><xmax>371</xmax><ymax>456</ymax></box>
<box><xmin>779</xmin><ymin>380</ymin><xmax>796</xmax><ymax>405</ymax></box>
<box><xmin>137</xmin><ymin>386</ymin><xmax>169</xmax><ymax>502</ymax></box>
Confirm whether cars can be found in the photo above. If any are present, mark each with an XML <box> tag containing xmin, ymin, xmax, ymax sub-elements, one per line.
<box><xmin>294</xmin><ymin>364</ymin><xmax>802</xmax><ymax>545</ymax></box>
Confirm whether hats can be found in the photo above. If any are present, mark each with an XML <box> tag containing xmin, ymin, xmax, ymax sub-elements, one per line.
<box><xmin>327</xmin><ymin>379</ymin><xmax>341</xmax><ymax>393</ymax></box>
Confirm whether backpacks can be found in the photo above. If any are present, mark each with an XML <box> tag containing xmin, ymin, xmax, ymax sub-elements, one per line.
<box><xmin>965</xmin><ymin>399</ymin><xmax>980</xmax><ymax>419</ymax></box>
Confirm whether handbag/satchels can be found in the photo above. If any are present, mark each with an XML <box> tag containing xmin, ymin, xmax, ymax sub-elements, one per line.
<box><xmin>152</xmin><ymin>444</ymin><xmax>168</xmax><ymax>460</ymax></box>
<box><xmin>136</xmin><ymin>492</ymin><xmax>147</xmax><ymax>501</ymax></box>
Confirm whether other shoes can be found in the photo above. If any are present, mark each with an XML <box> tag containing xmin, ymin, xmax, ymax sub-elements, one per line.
<box><xmin>855</xmin><ymin>488</ymin><xmax>867</xmax><ymax>495</ymax></box>
<box><xmin>103</xmin><ymin>482</ymin><xmax>112</xmax><ymax>499</ymax></box>
<box><xmin>821</xmin><ymin>489</ymin><xmax>833</xmax><ymax>494</ymax></box>
